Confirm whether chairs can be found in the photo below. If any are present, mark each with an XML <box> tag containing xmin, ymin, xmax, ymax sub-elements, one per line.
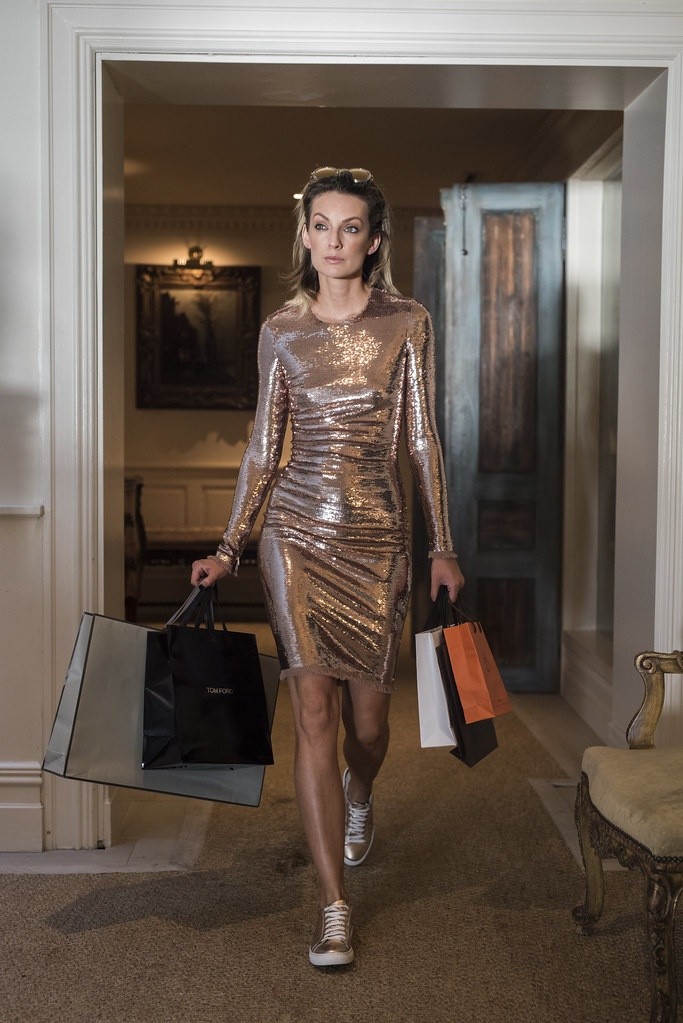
<box><xmin>573</xmin><ymin>651</ymin><xmax>683</xmax><ymax>1023</ymax></box>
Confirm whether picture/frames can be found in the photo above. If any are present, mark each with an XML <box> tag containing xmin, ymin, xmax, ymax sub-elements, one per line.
<box><xmin>136</xmin><ymin>246</ymin><xmax>261</xmax><ymax>411</ymax></box>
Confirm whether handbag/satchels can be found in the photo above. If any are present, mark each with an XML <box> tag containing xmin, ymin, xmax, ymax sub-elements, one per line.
<box><xmin>414</xmin><ymin>587</ymin><xmax>514</xmax><ymax>766</ymax></box>
<box><xmin>141</xmin><ymin>579</ymin><xmax>275</xmax><ymax>770</ymax></box>
<box><xmin>40</xmin><ymin>576</ymin><xmax>280</xmax><ymax>807</ymax></box>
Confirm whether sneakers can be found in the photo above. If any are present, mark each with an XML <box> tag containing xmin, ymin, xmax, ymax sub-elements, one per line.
<box><xmin>342</xmin><ymin>767</ymin><xmax>374</xmax><ymax>866</ymax></box>
<box><xmin>309</xmin><ymin>900</ymin><xmax>356</xmax><ymax>966</ymax></box>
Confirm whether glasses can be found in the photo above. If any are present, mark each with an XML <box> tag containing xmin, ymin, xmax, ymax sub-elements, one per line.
<box><xmin>309</xmin><ymin>166</ymin><xmax>373</xmax><ymax>185</ymax></box>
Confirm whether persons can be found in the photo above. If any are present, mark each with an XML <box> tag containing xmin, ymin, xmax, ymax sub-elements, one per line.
<box><xmin>191</xmin><ymin>166</ymin><xmax>466</xmax><ymax>966</ymax></box>
